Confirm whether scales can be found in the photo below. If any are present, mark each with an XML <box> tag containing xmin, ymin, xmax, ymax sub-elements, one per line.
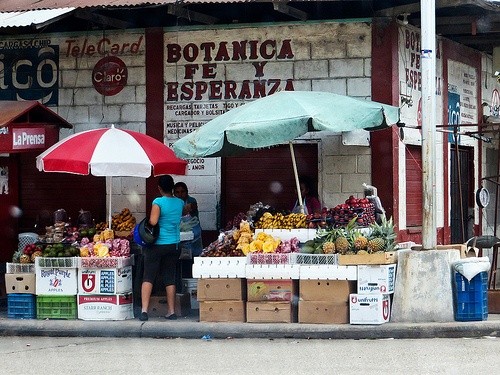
<box><xmin>468</xmin><ymin>186</ymin><xmax>500</xmax><ymax>248</ymax></box>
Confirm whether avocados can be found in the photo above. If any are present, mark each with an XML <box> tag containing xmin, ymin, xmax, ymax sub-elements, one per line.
<box><xmin>42</xmin><ymin>243</ymin><xmax>80</xmax><ymax>258</ymax></box>
<box><xmin>79</xmin><ymin>228</ymin><xmax>95</xmax><ymax>234</ymax></box>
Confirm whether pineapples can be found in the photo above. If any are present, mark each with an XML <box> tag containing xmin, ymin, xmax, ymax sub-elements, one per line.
<box><xmin>249</xmin><ymin>232</ymin><xmax>280</xmax><ymax>253</ymax></box>
<box><xmin>81</xmin><ymin>230</ymin><xmax>114</xmax><ymax>257</ymax></box>
<box><xmin>20</xmin><ymin>250</ymin><xmax>42</xmax><ymax>263</ymax></box>
<box><xmin>323</xmin><ymin>237</ymin><xmax>386</xmax><ymax>255</ymax></box>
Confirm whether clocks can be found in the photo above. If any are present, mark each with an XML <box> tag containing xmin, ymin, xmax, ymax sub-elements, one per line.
<box><xmin>476</xmin><ymin>187</ymin><xmax>490</xmax><ymax>208</ymax></box>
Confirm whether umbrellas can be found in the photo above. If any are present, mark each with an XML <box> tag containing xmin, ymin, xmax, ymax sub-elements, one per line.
<box><xmin>170</xmin><ymin>91</ymin><xmax>400</xmax><ymax>214</ymax></box>
<box><xmin>35</xmin><ymin>123</ymin><xmax>187</xmax><ymax>230</ymax></box>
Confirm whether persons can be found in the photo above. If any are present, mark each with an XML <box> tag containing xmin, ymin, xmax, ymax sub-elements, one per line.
<box><xmin>293</xmin><ymin>178</ymin><xmax>321</xmax><ymax>213</ymax></box>
<box><xmin>139</xmin><ymin>175</ymin><xmax>184</xmax><ymax>321</ymax></box>
<box><xmin>174</xmin><ymin>182</ymin><xmax>202</xmax><ymax>279</ymax></box>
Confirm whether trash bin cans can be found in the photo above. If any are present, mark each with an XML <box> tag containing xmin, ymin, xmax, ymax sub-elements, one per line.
<box><xmin>452</xmin><ymin>256</ymin><xmax>489</xmax><ymax>321</ymax></box>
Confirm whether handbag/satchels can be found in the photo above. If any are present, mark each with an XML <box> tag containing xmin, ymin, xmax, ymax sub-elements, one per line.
<box><xmin>178</xmin><ymin>247</ymin><xmax>192</xmax><ymax>260</ymax></box>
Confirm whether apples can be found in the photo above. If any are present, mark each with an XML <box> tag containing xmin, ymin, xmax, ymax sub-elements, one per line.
<box><xmin>307</xmin><ymin>195</ymin><xmax>375</xmax><ymax>229</ymax></box>
<box><xmin>67</xmin><ymin>227</ymin><xmax>80</xmax><ymax>241</ymax></box>
<box><xmin>23</xmin><ymin>243</ymin><xmax>42</xmax><ymax>255</ymax></box>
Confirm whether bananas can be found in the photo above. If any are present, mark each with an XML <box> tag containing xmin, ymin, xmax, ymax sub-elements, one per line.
<box><xmin>95</xmin><ymin>208</ymin><xmax>136</xmax><ymax>232</ymax></box>
<box><xmin>255</xmin><ymin>212</ymin><xmax>308</xmax><ymax>229</ymax></box>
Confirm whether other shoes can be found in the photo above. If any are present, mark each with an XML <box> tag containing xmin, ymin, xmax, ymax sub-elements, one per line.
<box><xmin>139</xmin><ymin>312</ymin><xmax>148</xmax><ymax>321</ymax></box>
<box><xmin>163</xmin><ymin>312</ymin><xmax>176</xmax><ymax>319</ymax></box>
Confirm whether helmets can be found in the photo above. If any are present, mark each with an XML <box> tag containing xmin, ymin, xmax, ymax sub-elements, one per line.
<box><xmin>133</xmin><ymin>216</ymin><xmax>160</xmax><ymax>248</ymax></box>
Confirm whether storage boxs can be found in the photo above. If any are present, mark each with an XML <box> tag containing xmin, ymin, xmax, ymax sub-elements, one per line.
<box><xmin>148</xmin><ymin>293</ymin><xmax>191</xmax><ymax>317</ymax></box>
<box><xmin>455</xmin><ymin>262</ymin><xmax>488</xmax><ymax>321</ymax></box>
<box><xmin>5</xmin><ymin>254</ymin><xmax>134</xmax><ymax>320</ymax></box>
<box><xmin>192</xmin><ymin>227</ymin><xmax>398</xmax><ymax>324</ymax></box>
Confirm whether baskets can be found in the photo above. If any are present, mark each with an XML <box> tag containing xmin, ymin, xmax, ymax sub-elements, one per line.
<box><xmin>35</xmin><ymin>256</ymin><xmax>78</xmax><ymax>269</ymax></box>
<box><xmin>296</xmin><ymin>252</ymin><xmax>339</xmax><ymax>266</ymax></box>
<box><xmin>5</xmin><ymin>261</ymin><xmax>35</xmax><ymax>273</ymax></box>
<box><xmin>78</xmin><ymin>253</ymin><xmax>135</xmax><ymax>268</ymax></box>
<box><xmin>249</xmin><ymin>252</ymin><xmax>295</xmax><ymax>265</ymax></box>
